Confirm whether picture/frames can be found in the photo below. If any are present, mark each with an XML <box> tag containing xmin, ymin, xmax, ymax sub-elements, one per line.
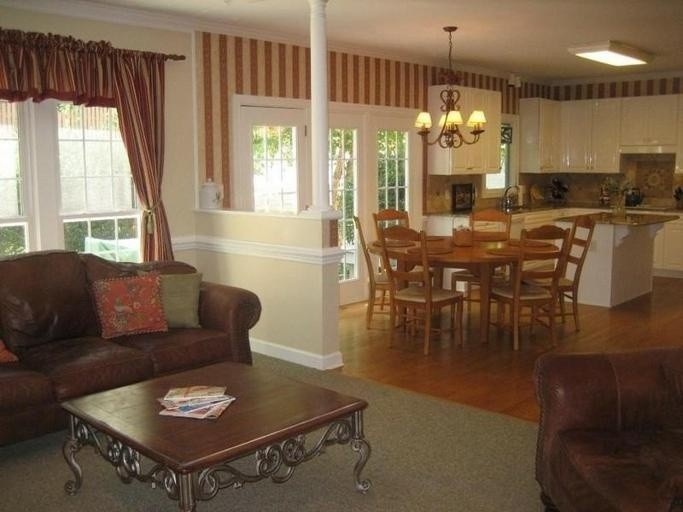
<box><xmin>452</xmin><ymin>183</ymin><xmax>473</xmax><ymax>213</ymax></box>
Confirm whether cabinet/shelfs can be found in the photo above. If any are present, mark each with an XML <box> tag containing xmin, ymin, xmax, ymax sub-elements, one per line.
<box><xmin>561</xmin><ymin>98</ymin><xmax>621</xmax><ymax>173</ymax></box>
<box><xmin>653</xmin><ymin>211</ymin><xmax>683</xmax><ymax>277</ymax></box>
<box><xmin>519</xmin><ymin>97</ymin><xmax>559</xmax><ymax>174</ymax></box>
<box><xmin>622</xmin><ymin>95</ymin><xmax>678</xmax><ymax>153</ymax></box>
<box><xmin>428</xmin><ymin>84</ymin><xmax>501</xmax><ymax>176</ymax></box>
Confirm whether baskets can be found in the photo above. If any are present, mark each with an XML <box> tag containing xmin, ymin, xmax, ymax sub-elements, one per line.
<box><xmin>451</xmin><ymin>214</ymin><xmax>474</xmax><ymax>247</ymax></box>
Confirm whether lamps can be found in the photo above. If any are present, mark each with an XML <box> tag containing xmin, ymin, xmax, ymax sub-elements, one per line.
<box><xmin>567</xmin><ymin>40</ymin><xmax>653</xmax><ymax>67</ymax></box>
<box><xmin>414</xmin><ymin>27</ymin><xmax>487</xmax><ymax>149</ymax></box>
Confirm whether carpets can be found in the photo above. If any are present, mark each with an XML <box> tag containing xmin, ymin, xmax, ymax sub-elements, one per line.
<box><xmin>0</xmin><ymin>352</ymin><xmax>545</xmax><ymax>512</ymax></box>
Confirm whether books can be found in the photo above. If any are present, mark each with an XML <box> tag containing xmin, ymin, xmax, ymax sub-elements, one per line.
<box><xmin>155</xmin><ymin>384</ymin><xmax>237</xmax><ymax>422</ymax></box>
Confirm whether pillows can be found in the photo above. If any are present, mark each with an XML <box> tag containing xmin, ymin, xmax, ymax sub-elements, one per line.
<box><xmin>0</xmin><ymin>339</ymin><xmax>20</xmax><ymax>363</ymax></box>
<box><xmin>136</xmin><ymin>269</ymin><xmax>205</xmax><ymax>328</ymax></box>
<box><xmin>88</xmin><ymin>268</ymin><xmax>169</xmax><ymax>340</ymax></box>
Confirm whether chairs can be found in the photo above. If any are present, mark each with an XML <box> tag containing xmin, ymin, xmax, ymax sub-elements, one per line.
<box><xmin>450</xmin><ymin>208</ymin><xmax>512</xmax><ymax>329</ymax></box>
<box><xmin>352</xmin><ymin>215</ymin><xmax>397</xmax><ymax>328</ymax></box>
<box><xmin>373</xmin><ymin>208</ymin><xmax>435</xmax><ymax>285</ymax></box>
<box><xmin>377</xmin><ymin>225</ymin><xmax>464</xmax><ymax>356</ymax></box>
<box><xmin>489</xmin><ymin>224</ymin><xmax>571</xmax><ymax>351</ymax></box>
<box><xmin>524</xmin><ymin>216</ymin><xmax>596</xmax><ymax>335</ymax></box>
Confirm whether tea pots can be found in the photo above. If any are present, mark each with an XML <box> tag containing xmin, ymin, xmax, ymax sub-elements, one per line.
<box><xmin>201</xmin><ymin>177</ymin><xmax>224</xmax><ymax>209</ymax></box>
<box><xmin>622</xmin><ymin>187</ymin><xmax>645</xmax><ymax>206</ymax></box>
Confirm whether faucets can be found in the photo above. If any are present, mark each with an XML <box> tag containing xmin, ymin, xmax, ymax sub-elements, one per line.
<box><xmin>501</xmin><ymin>185</ymin><xmax>521</xmax><ymax>208</ymax></box>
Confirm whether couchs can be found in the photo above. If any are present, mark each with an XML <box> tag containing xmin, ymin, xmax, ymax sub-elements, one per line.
<box><xmin>533</xmin><ymin>349</ymin><xmax>682</xmax><ymax>511</ymax></box>
<box><xmin>1</xmin><ymin>249</ymin><xmax>261</xmax><ymax>448</ymax></box>
